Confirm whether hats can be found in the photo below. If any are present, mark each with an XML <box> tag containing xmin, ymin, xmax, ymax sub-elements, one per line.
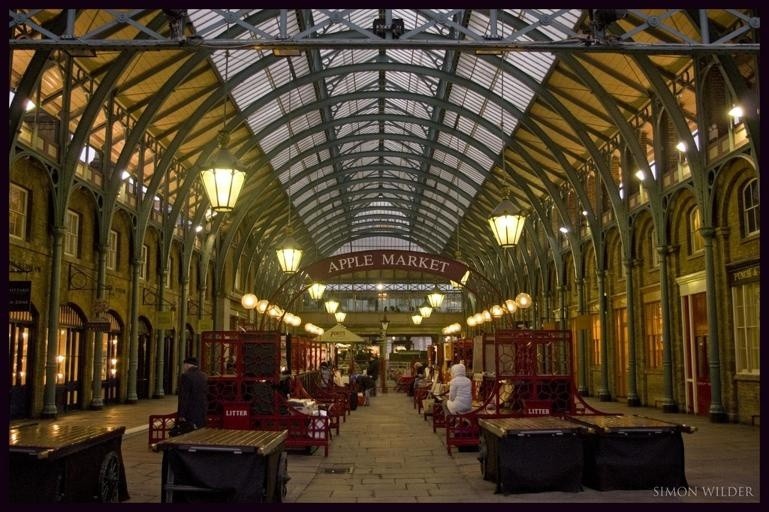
<box><xmin>184</xmin><ymin>358</ymin><xmax>199</xmax><ymax>365</ymax></box>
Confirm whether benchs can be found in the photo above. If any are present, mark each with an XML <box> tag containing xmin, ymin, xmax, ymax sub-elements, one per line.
<box><xmin>5</xmin><ymin>375</ymin><xmax>696</xmax><ymax>504</ymax></box>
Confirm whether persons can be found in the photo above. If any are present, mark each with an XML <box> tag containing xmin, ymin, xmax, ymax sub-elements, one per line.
<box><xmin>319</xmin><ymin>361</ymin><xmax>374</xmax><ymax>405</ymax></box>
<box><xmin>441</xmin><ymin>362</ymin><xmax>473</xmax><ymax>422</ymax></box>
<box><xmin>175</xmin><ymin>355</ymin><xmax>208</xmax><ymax>437</ymax></box>
<box><xmin>412</xmin><ymin>362</ymin><xmax>430</xmax><ymax>389</ymax></box>
<box><xmin>367</xmin><ymin>356</ymin><xmax>379</xmax><ymax>397</ymax></box>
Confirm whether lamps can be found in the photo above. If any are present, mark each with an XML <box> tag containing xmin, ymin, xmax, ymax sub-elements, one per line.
<box><xmin>242</xmin><ymin>293</ymin><xmax>348</xmax><ymax>337</ymax></box>
<box><xmin>308</xmin><ymin>171</ymin><xmax>326</xmax><ymax>301</ymax></box>
<box><xmin>411</xmin><ymin>291</ymin><xmax>532</xmax><ymax>342</ymax></box>
<box><xmin>272</xmin><ymin>58</ymin><xmax>303</xmax><ymax>274</ymax></box>
<box><xmin>199</xmin><ymin>10</ymin><xmax>249</xmax><ymax>213</ymax></box>
<box><xmin>488</xmin><ymin>8</ymin><xmax>527</xmax><ymax>248</ymax></box>
<box><xmin>449</xmin><ymin>119</ymin><xmax>470</xmax><ymax>289</ymax></box>
